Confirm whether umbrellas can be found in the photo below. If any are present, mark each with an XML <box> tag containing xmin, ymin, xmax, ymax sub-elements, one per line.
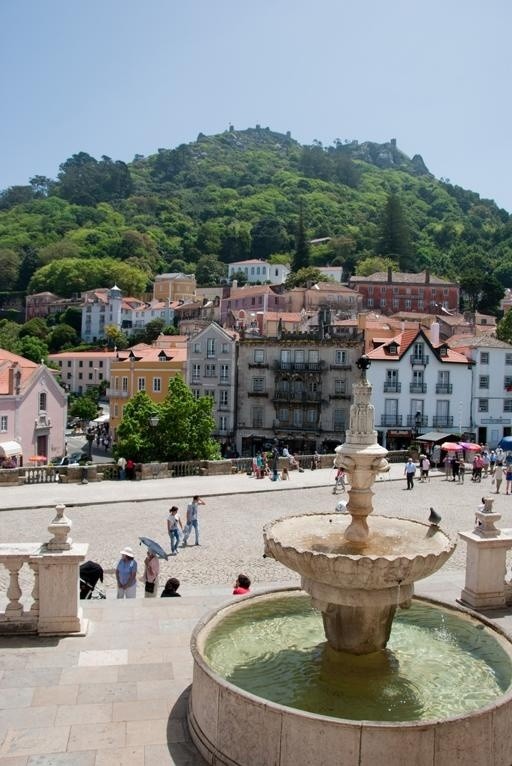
<box><xmin>139</xmin><ymin>536</ymin><xmax>167</xmax><ymax>561</ymax></box>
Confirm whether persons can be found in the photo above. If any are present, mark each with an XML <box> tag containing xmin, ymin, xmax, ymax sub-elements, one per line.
<box><xmin>115</xmin><ymin>454</ymin><xmax>127</xmax><ymax>480</ymax></box>
<box><xmin>127</xmin><ymin>458</ymin><xmax>137</xmax><ymax>479</ymax></box>
<box><xmin>137</xmin><ymin>547</ymin><xmax>160</xmax><ymax>598</ymax></box>
<box><xmin>474</xmin><ymin>496</ymin><xmax>486</xmax><ymax>527</ymax></box>
<box><xmin>252</xmin><ymin>443</ymin><xmax>328</xmax><ymax>481</ymax></box>
<box><xmin>167</xmin><ymin>505</ymin><xmax>184</xmax><ymax>555</ymax></box>
<box><xmin>183</xmin><ymin>495</ymin><xmax>206</xmax><ymax>547</ymax></box>
<box><xmin>115</xmin><ymin>547</ymin><xmax>137</xmax><ymax>600</ymax></box>
<box><xmin>161</xmin><ymin>578</ymin><xmax>181</xmax><ymax>598</ymax></box>
<box><xmin>232</xmin><ymin>574</ymin><xmax>250</xmax><ymax>595</ymax></box>
<box><xmin>73</xmin><ymin>419</ymin><xmax>111</xmax><ymax>453</ymax></box>
<box><xmin>332</xmin><ymin>467</ymin><xmax>346</xmax><ymax>495</ymax></box>
<box><xmin>403</xmin><ymin>436</ymin><xmax>512</xmax><ymax>495</ymax></box>
<box><xmin>1</xmin><ymin>456</ymin><xmax>16</xmax><ymax>468</ymax></box>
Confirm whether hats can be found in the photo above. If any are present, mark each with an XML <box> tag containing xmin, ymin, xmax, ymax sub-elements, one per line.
<box><xmin>120</xmin><ymin>547</ymin><xmax>135</xmax><ymax>558</ymax></box>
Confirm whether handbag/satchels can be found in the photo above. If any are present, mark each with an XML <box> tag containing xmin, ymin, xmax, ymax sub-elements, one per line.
<box><xmin>145</xmin><ymin>581</ymin><xmax>154</xmax><ymax>593</ymax></box>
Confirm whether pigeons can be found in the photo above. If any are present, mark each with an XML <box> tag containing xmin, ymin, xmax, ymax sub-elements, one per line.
<box><xmin>428</xmin><ymin>507</ymin><xmax>442</xmax><ymax>526</ymax></box>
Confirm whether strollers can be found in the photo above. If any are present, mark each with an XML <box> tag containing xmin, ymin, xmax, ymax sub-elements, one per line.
<box><xmin>79</xmin><ymin>561</ymin><xmax>106</xmax><ymax>600</ymax></box>
